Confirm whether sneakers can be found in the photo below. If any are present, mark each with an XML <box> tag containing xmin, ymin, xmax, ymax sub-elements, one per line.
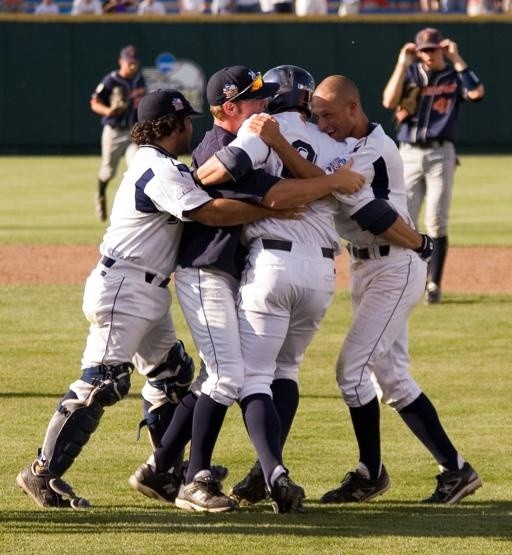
<box><xmin>424</xmin><ymin>279</ymin><xmax>440</xmax><ymax>307</ymax></box>
<box><xmin>421</xmin><ymin>464</ymin><xmax>485</xmax><ymax>507</ymax></box>
<box><xmin>95</xmin><ymin>193</ymin><xmax>106</xmax><ymax>220</ymax></box>
<box><xmin>16</xmin><ymin>457</ymin><xmax>90</xmax><ymax>509</ymax></box>
<box><xmin>129</xmin><ymin>463</ymin><xmax>181</xmax><ymax>501</ymax></box>
<box><xmin>229</xmin><ymin>475</ymin><xmax>305</xmax><ymax>514</ymax></box>
<box><xmin>322</xmin><ymin>460</ymin><xmax>391</xmax><ymax>504</ymax></box>
<box><xmin>182</xmin><ymin>459</ymin><xmax>228</xmax><ymax>487</ymax></box>
<box><xmin>176</xmin><ymin>480</ymin><xmax>235</xmax><ymax>514</ymax></box>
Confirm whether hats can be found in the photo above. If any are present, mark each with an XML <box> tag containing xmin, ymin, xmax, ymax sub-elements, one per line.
<box><xmin>414</xmin><ymin>27</ymin><xmax>443</xmax><ymax>52</ymax></box>
<box><xmin>206</xmin><ymin>67</ymin><xmax>281</xmax><ymax>104</ymax></box>
<box><xmin>119</xmin><ymin>45</ymin><xmax>142</xmax><ymax>63</ymax></box>
<box><xmin>139</xmin><ymin>88</ymin><xmax>203</xmax><ymax>119</ymax></box>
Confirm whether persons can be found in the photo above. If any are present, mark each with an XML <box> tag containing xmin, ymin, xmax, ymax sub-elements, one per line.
<box><xmin>2</xmin><ymin>1</ymin><xmax>511</xmax><ymax>19</ymax></box>
<box><xmin>128</xmin><ymin>62</ymin><xmax>366</xmax><ymax>513</ymax></box>
<box><xmin>195</xmin><ymin>64</ymin><xmax>434</xmax><ymax>513</ymax></box>
<box><xmin>14</xmin><ymin>86</ymin><xmax>310</xmax><ymax>512</ymax></box>
<box><xmin>245</xmin><ymin>72</ymin><xmax>484</xmax><ymax>504</ymax></box>
<box><xmin>381</xmin><ymin>26</ymin><xmax>486</xmax><ymax>303</ymax></box>
<box><xmin>88</xmin><ymin>45</ymin><xmax>146</xmax><ymax>222</ymax></box>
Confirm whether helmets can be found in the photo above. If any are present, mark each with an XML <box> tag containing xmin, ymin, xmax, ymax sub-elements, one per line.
<box><xmin>263</xmin><ymin>65</ymin><xmax>315</xmax><ymax>116</ymax></box>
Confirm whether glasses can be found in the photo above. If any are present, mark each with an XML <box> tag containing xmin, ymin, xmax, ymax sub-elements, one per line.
<box><xmin>224</xmin><ymin>69</ymin><xmax>263</xmax><ymax>102</ymax></box>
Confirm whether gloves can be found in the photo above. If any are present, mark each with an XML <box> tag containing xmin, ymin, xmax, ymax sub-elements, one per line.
<box><xmin>414</xmin><ymin>231</ymin><xmax>434</xmax><ymax>258</ymax></box>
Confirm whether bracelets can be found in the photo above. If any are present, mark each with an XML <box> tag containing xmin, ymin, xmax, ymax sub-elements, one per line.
<box><xmin>459</xmin><ymin>67</ymin><xmax>481</xmax><ymax>93</ymax></box>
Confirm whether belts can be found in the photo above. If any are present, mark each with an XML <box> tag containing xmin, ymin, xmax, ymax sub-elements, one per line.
<box><xmin>99</xmin><ymin>254</ymin><xmax>172</xmax><ymax>293</ymax></box>
<box><xmin>259</xmin><ymin>236</ymin><xmax>335</xmax><ymax>262</ymax></box>
<box><xmin>349</xmin><ymin>245</ymin><xmax>408</xmax><ymax>259</ymax></box>
